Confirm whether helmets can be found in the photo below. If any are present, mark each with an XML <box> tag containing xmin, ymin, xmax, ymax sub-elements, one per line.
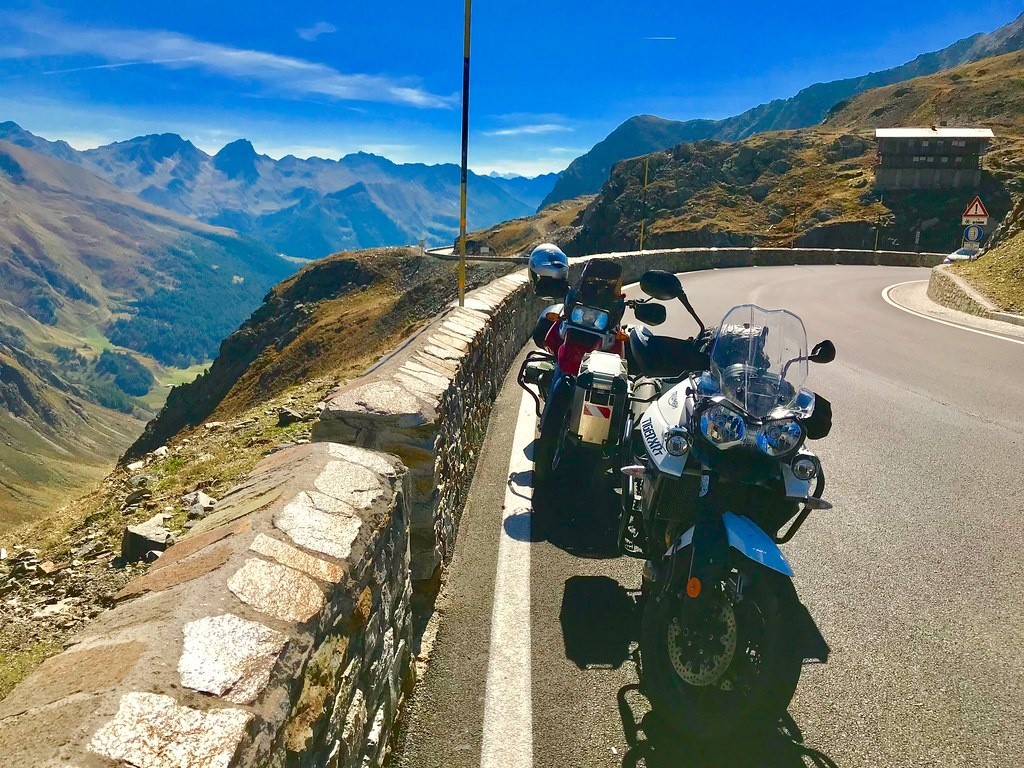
<box><xmin>528</xmin><ymin>242</ymin><xmax>570</xmax><ymax>297</ymax></box>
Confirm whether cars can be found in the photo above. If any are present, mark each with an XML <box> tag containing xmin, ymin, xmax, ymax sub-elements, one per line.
<box><xmin>942</xmin><ymin>247</ymin><xmax>984</xmax><ymax>266</ymax></box>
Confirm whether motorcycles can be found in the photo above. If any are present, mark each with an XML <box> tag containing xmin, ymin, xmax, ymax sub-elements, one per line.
<box><xmin>517</xmin><ymin>257</ymin><xmax>667</xmax><ymax>487</ymax></box>
<box><xmin>599</xmin><ymin>269</ymin><xmax>836</xmax><ymax>760</ymax></box>
